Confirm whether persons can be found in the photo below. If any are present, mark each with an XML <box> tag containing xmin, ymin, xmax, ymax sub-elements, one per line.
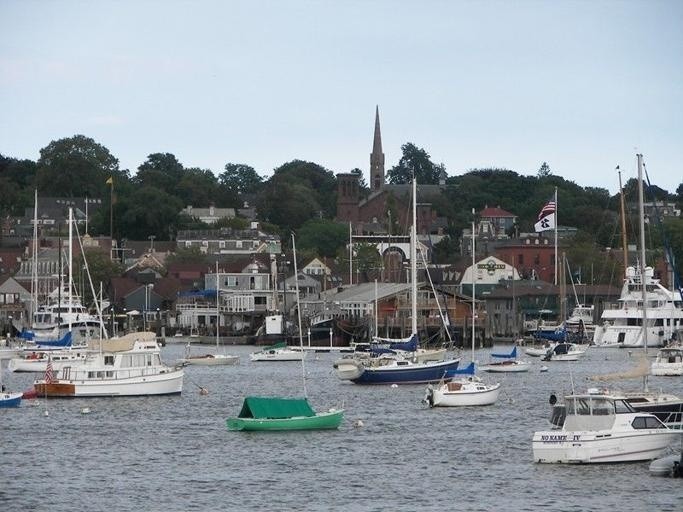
<box><xmin>30</xmin><ymin>352</ymin><xmax>38</xmax><ymax>359</ymax></box>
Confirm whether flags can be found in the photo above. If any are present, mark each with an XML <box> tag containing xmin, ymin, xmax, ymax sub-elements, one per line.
<box><xmin>535</xmin><ymin>191</ymin><xmax>556</xmax><ymax>233</ymax></box>
<box><xmin>44</xmin><ymin>354</ymin><xmax>53</xmax><ymax>384</ymax></box>
